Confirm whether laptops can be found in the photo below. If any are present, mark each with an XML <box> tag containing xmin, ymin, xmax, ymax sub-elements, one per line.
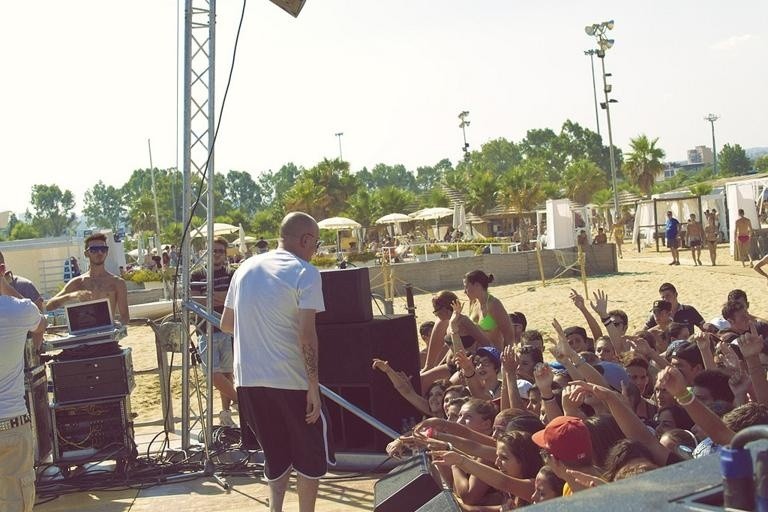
<box><xmin>65</xmin><ymin>298</ymin><xmax>114</xmax><ymax>335</ymax></box>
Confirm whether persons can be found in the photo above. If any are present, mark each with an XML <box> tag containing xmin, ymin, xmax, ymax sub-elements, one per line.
<box><xmin>190</xmin><ymin>236</ymin><xmax>239</xmax><ymax>429</ymax></box>
<box><xmin>219</xmin><ymin>211</ymin><xmax>337</xmax><ymax>512</ymax></box>
<box><xmin>45</xmin><ymin>235</ymin><xmax>131</xmax><ymax>326</ymax></box>
<box><xmin>360</xmin><ymin>233</ymin><xmax>415</xmax><ymax>265</ymax></box>
<box><xmin>0</xmin><ymin>253</ymin><xmax>44</xmax><ymax>339</ymax></box>
<box><xmin>0</xmin><ymin>275</ymin><xmax>50</xmax><ymax>511</ymax></box>
<box><xmin>665</xmin><ymin>197</ymin><xmax>768</xmax><ymax>281</ymax></box>
<box><xmin>371</xmin><ymin>268</ymin><xmax>767</xmax><ymax>511</ymax></box>
<box><xmin>494</xmin><ymin>219</ymin><xmax>547</xmax><ymax>250</ymax></box>
<box><xmin>231</xmin><ymin>235</ymin><xmax>270</xmax><ymax>262</ymax></box>
<box><xmin>576</xmin><ymin>209</ymin><xmax>633</xmax><ymax>261</ymax></box>
<box><xmin>117</xmin><ymin>243</ymin><xmax>179</xmax><ymax>277</ymax></box>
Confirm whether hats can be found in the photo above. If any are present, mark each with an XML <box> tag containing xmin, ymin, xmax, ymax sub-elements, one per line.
<box><xmin>672</xmin><ymin>342</ymin><xmax>699</xmax><ymax>360</ymax></box>
<box><xmin>531</xmin><ymin>416</ymin><xmax>592</xmax><ymax>462</ymax></box>
<box><xmin>711</xmin><ymin>332</ymin><xmax>738</xmax><ymax>343</ymax></box>
<box><xmin>492</xmin><ymin>379</ymin><xmax>532</xmax><ymax>401</ymax></box>
<box><xmin>595</xmin><ymin>362</ymin><xmax>629</xmax><ymax>392</ymax></box>
<box><xmin>478</xmin><ymin>347</ymin><xmax>500</xmax><ymax>369</ymax></box>
<box><xmin>650</xmin><ymin>300</ymin><xmax>672</xmax><ymax>312</ymax></box>
<box><xmin>564</xmin><ymin>326</ymin><xmax>587</xmax><ymax>343</ymax></box>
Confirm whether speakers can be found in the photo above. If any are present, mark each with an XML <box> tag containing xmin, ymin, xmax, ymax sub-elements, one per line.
<box><xmin>373</xmin><ymin>453</ymin><xmax>442</xmax><ymax>511</ymax></box>
<box><xmin>416</xmin><ymin>489</ymin><xmax>463</xmax><ymax>512</ymax></box>
<box><xmin>316</xmin><ymin>266</ymin><xmax>373</xmax><ymax>325</ymax></box>
<box><xmin>317</xmin><ymin>315</ymin><xmax>421</xmax><ymax>382</ymax></box>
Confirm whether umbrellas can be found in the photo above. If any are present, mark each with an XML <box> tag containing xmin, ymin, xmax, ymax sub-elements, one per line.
<box><xmin>375</xmin><ymin>213</ymin><xmax>412</xmax><ymax>234</ymax></box>
<box><xmin>411</xmin><ymin>202</ymin><xmax>467</xmax><ymax>243</ymax></box>
<box><xmin>136</xmin><ymin>236</ymin><xmax>146</xmax><ymax>270</ymax></box>
<box><xmin>316</xmin><ymin>216</ymin><xmax>363</xmax><ymax>251</ymax></box>
<box><xmin>190</xmin><ymin>222</ymin><xmax>249</xmax><ymax>260</ymax></box>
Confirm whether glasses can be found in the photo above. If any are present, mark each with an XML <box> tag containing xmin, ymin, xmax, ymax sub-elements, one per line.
<box><xmin>86</xmin><ymin>246</ymin><xmax>109</xmax><ymax>253</ymax></box>
<box><xmin>472</xmin><ymin>359</ymin><xmax>489</xmax><ymax>367</ymax></box>
<box><xmin>213</xmin><ymin>248</ymin><xmax>225</xmax><ymax>255</ymax></box>
<box><xmin>432</xmin><ymin>306</ymin><xmax>443</xmax><ymax>317</ymax></box>
<box><xmin>311</xmin><ymin>235</ymin><xmax>325</xmax><ymax>248</ymax></box>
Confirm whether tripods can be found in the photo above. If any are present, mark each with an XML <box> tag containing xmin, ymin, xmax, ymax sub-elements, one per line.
<box><xmin>154</xmin><ymin>255</ymin><xmax>262</xmax><ymax>491</ymax></box>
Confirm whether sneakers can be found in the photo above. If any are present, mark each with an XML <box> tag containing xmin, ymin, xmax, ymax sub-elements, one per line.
<box><xmin>668</xmin><ymin>260</ymin><xmax>754</xmax><ymax>268</ymax></box>
<box><xmin>219</xmin><ymin>410</ymin><xmax>238</xmax><ymax>428</ymax></box>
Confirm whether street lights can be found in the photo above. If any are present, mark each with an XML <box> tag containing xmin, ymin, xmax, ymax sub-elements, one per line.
<box><xmin>335</xmin><ymin>131</ymin><xmax>346</xmax><ymax>160</ymax></box>
<box><xmin>583</xmin><ymin>18</ymin><xmax>618</xmax><ymax>219</ymax></box>
<box><xmin>702</xmin><ymin>112</ymin><xmax>722</xmax><ymax>176</ymax></box>
<box><xmin>454</xmin><ymin>111</ymin><xmax>473</xmax><ymax>163</ymax></box>
